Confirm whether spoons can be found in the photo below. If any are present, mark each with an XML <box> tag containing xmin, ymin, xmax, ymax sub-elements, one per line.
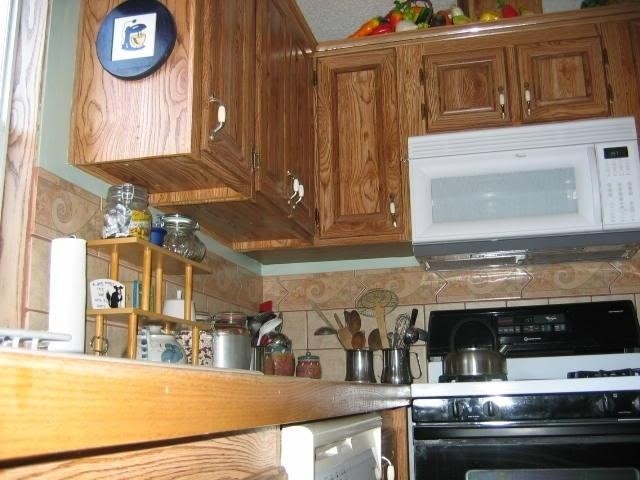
<box><xmin>387</xmin><ymin>332</ymin><xmax>400</xmax><ymax>348</ymax></box>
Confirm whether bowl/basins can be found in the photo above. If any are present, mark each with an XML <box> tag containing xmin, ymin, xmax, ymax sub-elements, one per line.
<box><xmin>150</xmin><ymin>227</ymin><xmax>167</xmax><ymax>245</ymax></box>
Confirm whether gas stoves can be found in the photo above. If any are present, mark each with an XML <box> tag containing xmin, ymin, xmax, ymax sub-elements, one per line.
<box><xmin>410</xmin><ymin>367</ymin><xmax>640</xmax><ymax>398</ymax></box>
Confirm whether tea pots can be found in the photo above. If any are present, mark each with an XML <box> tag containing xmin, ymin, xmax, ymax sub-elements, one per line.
<box><xmin>443</xmin><ymin>316</ymin><xmax>513</xmax><ymax>376</ymax></box>
<box><xmin>134</xmin><ymin>323</ymin><xmax>188</xmax><ymax>364</ymax></box>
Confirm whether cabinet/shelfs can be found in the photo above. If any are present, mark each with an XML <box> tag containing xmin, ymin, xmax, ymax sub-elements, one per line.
<box><xmin>0</xmin><ymin>426</ymin><xmax>288</xmax><ymax>480</ymax></box>
<box><xmin>69</xmin><ymin>0</ymin><xmax>252</xmax><ymax>194</ymax></box>
<box><xmin>414</xmin><ymin>20</ymin><xmax>608</xmax><ymax>135</ymax></box>
<box><xmin>140</xmin><ymin>2</ymin><xmax>317</xmax><ymax>243</ymax></box>
<box><xmin>316</xmin><ymin>41</ymin><xmax>412</xmax><ymax>259</ymax></box>
<box><xmin>83</xmin><ymin>237</ymin><xmax>212</xmax><ymax>365</ymax></box>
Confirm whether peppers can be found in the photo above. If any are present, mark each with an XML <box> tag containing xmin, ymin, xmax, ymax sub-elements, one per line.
<box><xmin>344</xmin><ymin>6</ymin><xmax>402</xmax><ymax>40</ymax></box>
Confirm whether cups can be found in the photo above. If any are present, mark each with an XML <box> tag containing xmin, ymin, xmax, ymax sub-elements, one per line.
<box><xmin>90</xmin><ymin>279</ymin><xmax>126</xmax><ymax>309</ymax></box>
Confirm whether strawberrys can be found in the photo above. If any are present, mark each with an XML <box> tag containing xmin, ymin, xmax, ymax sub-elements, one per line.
<box><xmin>501</xmin><ymin>5</ymin><xmax>519</xmax><ymax>19</ymax></box>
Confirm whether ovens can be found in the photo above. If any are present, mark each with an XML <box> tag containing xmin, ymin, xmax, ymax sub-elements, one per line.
<box><xmin>410</xmin><ymin>391</ymin><xmax>640</xmax><ymax>480</ymax></box>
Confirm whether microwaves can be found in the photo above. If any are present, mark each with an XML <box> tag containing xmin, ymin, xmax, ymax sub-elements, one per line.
<box><xmin>407</xmin><ymin>114</ymin><xmax>640</xmax><ymax>259</ymax></box>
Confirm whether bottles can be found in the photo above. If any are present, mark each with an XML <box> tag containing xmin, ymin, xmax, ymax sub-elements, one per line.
<box><xmin>160</xmin><ymin>213</ymin><xmax>207</xmax><ymax>263</ymax></box>
<box><xmin>263</xmin><ymin>344</ymin><xmax>295</xmax><ymax>376</ymax></box>
<box><xmin>295</xmin><ymin>351</ymin><xmax>322</xmax><ymax>379</ymax></box>
<box><xmin>212</xmin><ymin>312</ymin><xmax>252</xmax><ymax>370</ymax></box>
<box><xmin>171</xmin><ymin>311</ymin><xmax>214</xmax><ymax>369</ymax></box>
<box><xmin>100</xmin><ymin>183</ymin><xmax>152</xmax><ymax>241</ymax></box>
